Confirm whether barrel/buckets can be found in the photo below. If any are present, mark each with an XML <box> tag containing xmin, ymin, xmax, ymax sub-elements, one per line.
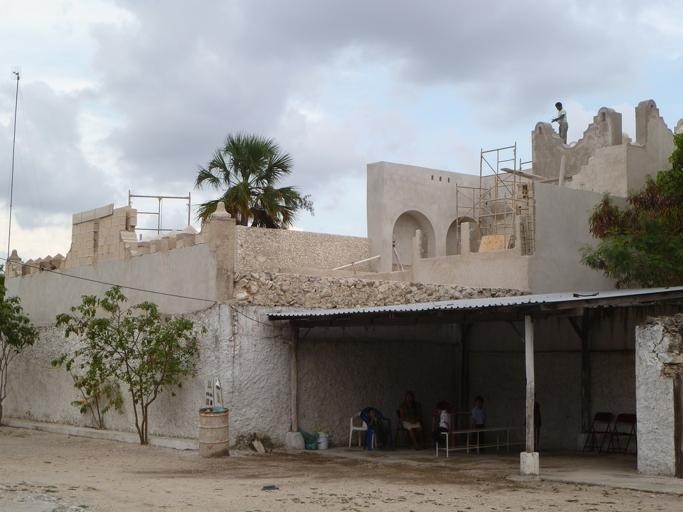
<box><xmin>316</xmin><ymin>431</ymin><xmax>330</xmax><ymax>449</ymax></box>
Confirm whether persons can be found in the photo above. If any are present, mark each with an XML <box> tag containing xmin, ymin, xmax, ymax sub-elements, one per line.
<box><xmin>430</xmin><ymin>399</ymin><xmax>451</xmax><ymax>448</ymax></box>
<box><xmin>471</xmin><ymin>395</ymin><xmax>487</xmax><ymax>443</ymax></box>
<box><xmin>366</xmin><ymin>408</ymin><xmax>383</xmax><ymax>451</ymax></box>
<box><xmin>399</xmin><ymin>390</ymin><xmax>428</xmax><ymax>450</ymax></box>
<box><xmin>551</xmin><ymin>101</ymin><xmax>569</xmax><ymax>144</ymax></box>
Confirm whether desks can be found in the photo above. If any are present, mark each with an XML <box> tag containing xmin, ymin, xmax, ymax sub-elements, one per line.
<box><xmin>436</xmin><ymin>426</ymin><xmax>523</xmax><ymax>458</ymax></box>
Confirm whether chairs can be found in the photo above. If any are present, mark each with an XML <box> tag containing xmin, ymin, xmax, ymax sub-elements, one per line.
<box><xmin>581</xmin><ymin>412</ymin><xmax>614</xmax><ymax>454</ymax></box>
<box><xmin>349</xmin><ymin>399</ymin><xmax>417</xmax><ymax>451</ymax></box>
<box><xmin>607</xmin><ymin>414</ymin><xmax>637</xmax><ymax>456</ymax></box>
